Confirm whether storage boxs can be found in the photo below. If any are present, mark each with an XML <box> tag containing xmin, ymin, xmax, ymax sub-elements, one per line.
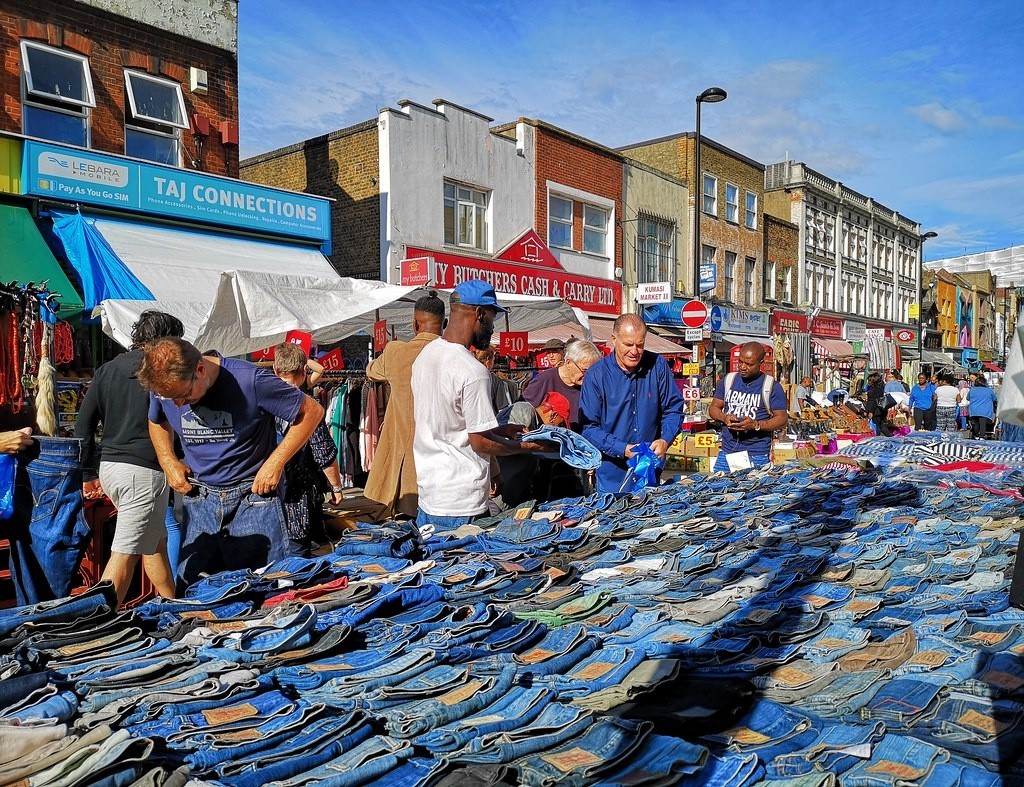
<box><xmin>667</xmin><ymin>434</ymin><xmax>722</xmax><ymax>474</ymax></box>
<box><xmin>772</xmin><ymin>427</ymin><xmax>874</xmax><ymax>464</ymax></box>
<box><xmin>908</xmin><ymin>417</ymin><xmax>915</xmax><ymax>425</ymax></box>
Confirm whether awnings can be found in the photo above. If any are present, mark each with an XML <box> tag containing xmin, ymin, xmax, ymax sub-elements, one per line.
<box><xmin>0</xmin><ymin>205</ymin><xmax>85</xmax><ymax>321</ymax></box>
<box><xmin>982</xmin><ymin>362</ymin><xmax>1003</xmax><ymax>372</ymax></box>
<box><xmin>813</xmin><ymin>339</ymin><xmax>865</xmax><ymax>376</ymax></box>
<box><xmin>722</xmin><ymin>335</ymin><xmax>774</xmax><ymax>349</ymax></box>
<box><xmin>47</xmin><ymin>209</ymin><xmax>594</xmax><ymax>375</ymax></box>
<box><xmin>489</xmin><ymin>318</ymin><xmax>694</xmax><ymax>355</ymax></box>
<box><xmin>901</xmin><ymin>347</ymin><xmax>962</xmax><ymax>366</ymax></box>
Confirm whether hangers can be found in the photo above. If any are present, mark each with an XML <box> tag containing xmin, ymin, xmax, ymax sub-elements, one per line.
<box><xmin>316</xmin><ymin>370</ymin><xmax>376</xmax><ymax>394</ymax></box>
<box><xmin>494</xmin><ymin>365</ymin><xmax>539</xmax><ymax>379</ymax></box>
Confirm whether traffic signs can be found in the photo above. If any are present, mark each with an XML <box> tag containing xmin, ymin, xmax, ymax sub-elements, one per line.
<box><xmin>709</xmin><ymin>305</ymin><xmax>768</xmax><ymax>336</ymax></box>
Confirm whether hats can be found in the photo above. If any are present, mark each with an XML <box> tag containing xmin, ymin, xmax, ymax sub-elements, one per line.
<box><xmin>540</xmin><ymin>339</ymin><xmax>565</xmax><ymax>350</ymax></box>
<box><xmin>449</xmin><ymin>280</ymin><xmax>509</xmax><ymax>312</ymax></box>
<box><xmin>541</xmin><ymin>392</ymin><xmax>571</xmax><ymax>429</ymax></box>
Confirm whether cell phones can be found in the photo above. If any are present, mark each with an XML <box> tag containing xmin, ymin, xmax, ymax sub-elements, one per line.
<box><xmin>730</xmin><ymin>420</ymin><xmax>741</xmax><ymax>427</ymax></box>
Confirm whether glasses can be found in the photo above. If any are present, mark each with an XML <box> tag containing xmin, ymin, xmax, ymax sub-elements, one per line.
<box><xmin>570</xmin><ymin>358</ymin><xmax>585</xmax><ymax>374</ymax></box>
<box><xmin>154</xmin><ymin>372</ymin><xmax>194</xmax><ymax>401</ymax></box>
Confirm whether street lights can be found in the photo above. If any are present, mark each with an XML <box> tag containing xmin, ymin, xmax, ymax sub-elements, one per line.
<box><xmin>1003</xmin><ymin>285</ymin><xmax>1017</xmax><ymax>371</ymax></box>
<box><xmin>917</xmin><ymin>232</ymin><xmax>938</xmax><ymax>359</ymax></box>
<box><xmin>693</xmin><ymin>86</ymin><xmax>727</xmax><ymax>297</ymax></box>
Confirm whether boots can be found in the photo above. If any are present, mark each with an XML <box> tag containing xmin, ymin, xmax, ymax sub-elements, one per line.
<box><xmin>772</xmin><ymin>403</ymin><xmax>874</xmax><ymax>442</ymax></box>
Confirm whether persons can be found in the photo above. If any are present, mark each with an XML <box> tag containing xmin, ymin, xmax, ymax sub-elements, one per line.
<box><xmin>863</xmin><ymin>372</ymin><xmax>894</xmax><ymax>437</ymax></box>
<box><xmin>708</xmin><ymin>342</ymin><xmax>789</xmax><ymax>473</ymax></box>
<box><xmin>470</xmin><ymin>338</ymin><xmax>600</xmax><ymax>504</ymax></box>
<box><xmin>884</xmin><ymin>374</ymin><xmax>910</xmax><ymax>394</ymax></box>
<box><xmin>0</xmin><ymin>427</ymin><xmax>34</xmax><ymax>455</ymax></box>
<box><xmin>792</xmin><ymin>377</ymin><xmax>819</xmax><ymax>413</ymax></box>
<box><xmin>133</xmin><ymin>334</ymin><xmax>326</xmax><ymax>579</ymax></box>
<box><xmin>71</xmin><ymin>308</ymin><xmax>186</xmax><ymax>611</ymax></box>
<box><xmin>576</xmin><ymin>314</ymin><xmax>684</xmax><ymax>498</ymax></box>
<box><xmin>410</xmin><ymin>278</ymin><xmax>562</xmax><ymax>528</ymax></box>
<box><xmin>907</xmin><ymin>373</ymin><xmax>1002</xmax><ymax>443</ymax></box>
<box><xmin>269</xmin><ymin>340</ymin><xmax>346</xmax><ymax>560</ymax></box>
<box><xmin>363</xmin><ymin>288</ymin><xmax>447</xmax><ymax>519</ymax></box>
<box><xmin>161</xmin><ymin>488</ymin><xmax>182</xmax><ymax>582</ymax></box>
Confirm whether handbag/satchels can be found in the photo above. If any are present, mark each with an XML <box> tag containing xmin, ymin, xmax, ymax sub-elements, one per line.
<box><xmin>876</xmin><ymin>393</ymin><xmax>897</xmax><ymax>411</ymax></box>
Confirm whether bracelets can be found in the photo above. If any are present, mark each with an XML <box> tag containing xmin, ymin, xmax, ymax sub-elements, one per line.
<box><xmin>331</xmin><ymin>485</ymin><xmax>342</xmax><ymax>492</ymax></box>
<box><xmin>753</xmin><ymin>420</ymin><xmax>761</xmax><ymax>430</ymax></box>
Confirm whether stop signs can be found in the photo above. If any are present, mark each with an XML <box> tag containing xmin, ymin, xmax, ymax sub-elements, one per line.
<box><xmin>680</xmin><ymin>301</ymin><xmax>708</xmax><ymax>329</ymax></box>
